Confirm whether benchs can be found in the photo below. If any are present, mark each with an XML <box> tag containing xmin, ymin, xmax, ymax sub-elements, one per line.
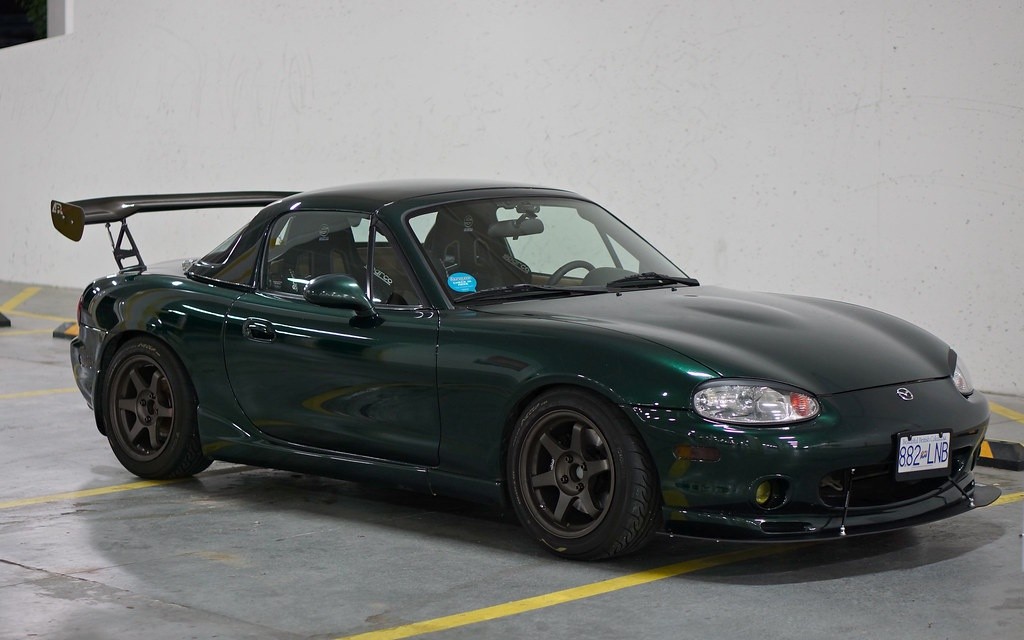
<box><xmin>273</xmin><ymin>245</ymin><xmax>423</xmax><ymax>303</ymax></box>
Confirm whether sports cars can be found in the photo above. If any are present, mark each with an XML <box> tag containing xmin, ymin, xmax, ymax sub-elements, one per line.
<box><xmin>48</xmin><ymin>178</ymin><xmax>1001</xmax><ymax>564</ymax></box>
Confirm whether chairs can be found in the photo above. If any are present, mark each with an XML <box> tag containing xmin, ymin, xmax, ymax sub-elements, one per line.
<box><xmin>423</xmin><ymin>207</ymin><xmax>529</xmax><ymax>298</ymax></box>
<box><xmin>278</xmin><ymin>216</ymin><xmax>391</xmax><ymax>302</ymax></box>
